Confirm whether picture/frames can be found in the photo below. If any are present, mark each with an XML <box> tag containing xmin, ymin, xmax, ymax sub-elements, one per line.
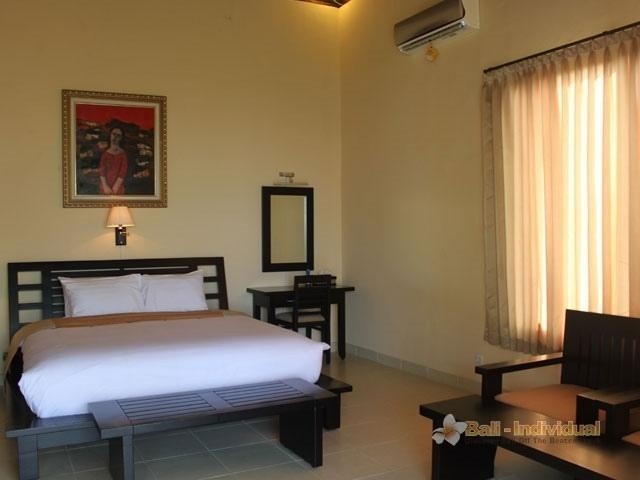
<box><xmin>61</xmin><ymin>89</ymin><xmax>168</xmax><ymax>209</ymax></box>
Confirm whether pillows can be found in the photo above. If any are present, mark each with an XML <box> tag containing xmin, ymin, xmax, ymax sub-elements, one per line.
<box><xmin>57</xmin><ymin>273</ymin><xmax>142</xmax><ymax>318</ymax></box>
<box><xmin>142</xmin><ymin>269</ymin><xmax>209</xmax><ymax>312</ymax></box>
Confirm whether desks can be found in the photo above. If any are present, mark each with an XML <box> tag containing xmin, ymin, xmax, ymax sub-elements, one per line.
<box><xmin>419</xmin><ymin>394</ymin><xmax>640</xmax><ymax>480</ymax></box>
<box><xmin>246</xmin><ymin>285</ymin><xmax>355</xmax><ymax>361</ymax></box>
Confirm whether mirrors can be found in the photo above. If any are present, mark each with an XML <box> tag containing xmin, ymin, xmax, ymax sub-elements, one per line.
<box><xmin>261</xmin><ymin>186</ymin><xmax>314</xmax><ymax>273</ymax></box>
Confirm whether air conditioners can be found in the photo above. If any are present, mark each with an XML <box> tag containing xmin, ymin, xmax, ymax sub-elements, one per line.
<box><xmin>393</xmin><ymin>0</ymin><xmax>481</xmax><ymax>56</ymax></box>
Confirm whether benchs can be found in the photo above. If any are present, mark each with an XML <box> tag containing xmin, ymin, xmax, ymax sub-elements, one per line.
<box><xmin>86</xmin><ymin>377</ymin><xmax>338</xmax><ymax>480</ymax></box>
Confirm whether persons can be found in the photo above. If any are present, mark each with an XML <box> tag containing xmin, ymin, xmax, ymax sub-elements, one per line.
<box><xmin>99</xmin><ymin>126</ymin><xmax>129</xmax><ymax>195</ymax></box>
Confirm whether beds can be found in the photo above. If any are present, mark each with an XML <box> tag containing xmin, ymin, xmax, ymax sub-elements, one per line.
<box><xmin>0</xmin><ymin>257</ymin><xmax>353</xmax><ymax>480</ymax></box>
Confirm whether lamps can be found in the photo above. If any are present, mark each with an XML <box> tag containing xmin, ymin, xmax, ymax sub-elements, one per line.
<box><xmin>104</xmin><ymin>206</ymin><xmax>136</xmax><ymax>245</ymax></box>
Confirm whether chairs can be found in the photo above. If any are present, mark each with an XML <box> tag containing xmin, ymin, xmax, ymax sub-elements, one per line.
<box><xmin>474</xmin><ymin>309</ymin><xmax>640</xmax><ymax>439</ymax></box>
<box><xmin>595</xmin><ymin>389</ymin><xmax>640</xmax><ymax>451</ymax></box>
<box><xmin>274</xmin><ymin>274</ymin><xmax>332</xmax><ymax>365</ymax></box>
